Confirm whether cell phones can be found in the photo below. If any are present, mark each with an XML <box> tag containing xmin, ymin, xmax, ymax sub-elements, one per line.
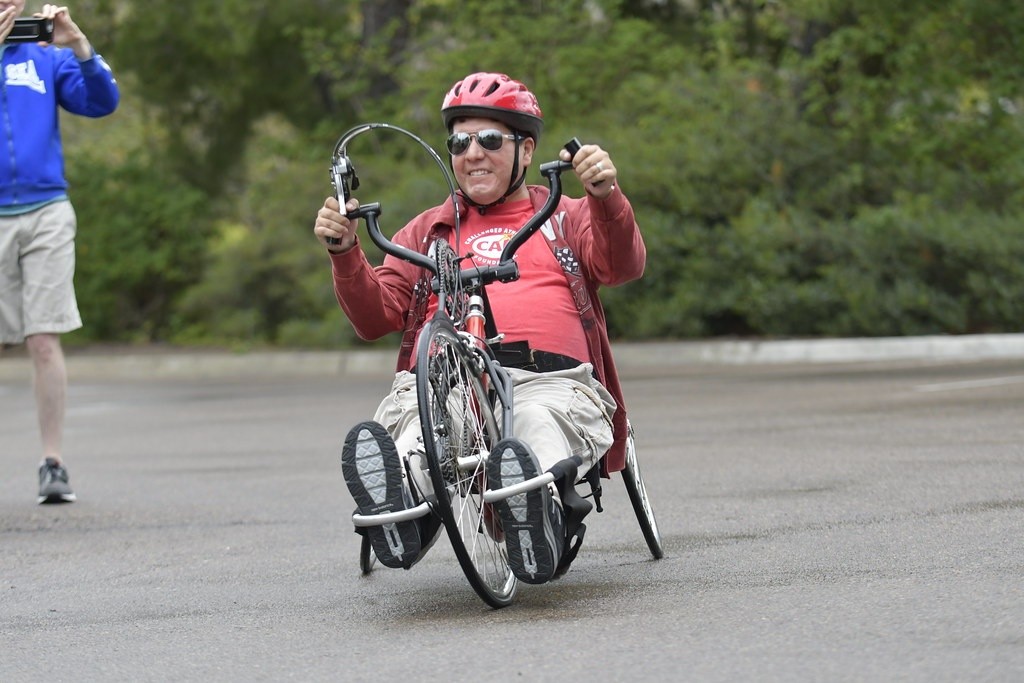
<box><xmin>4</xmin><ymin>17</ymin><xmax>55</xmax><ymax>44</ymax></box>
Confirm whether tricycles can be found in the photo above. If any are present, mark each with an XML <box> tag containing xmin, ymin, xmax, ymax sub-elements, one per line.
<box><xmin>325</xmin><ymin>122</ymin><xmax>666</xmax><ymax>611</ymax></box>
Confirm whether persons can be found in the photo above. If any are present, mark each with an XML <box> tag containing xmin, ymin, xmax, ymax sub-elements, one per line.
<box><xmin>0</xmin><ymin>0</ymin><xmax>120</xmax><ymax>503</ymax></box>
<box><xmin>314</xmin><ymin>71</ymin><xmax>646</xmax><ymax>585</ymax></box>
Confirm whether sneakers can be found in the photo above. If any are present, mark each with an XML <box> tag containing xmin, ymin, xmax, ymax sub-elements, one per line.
<box><xmin>487</xmin><ymin>439</ymin><xmax>566</xmax><ymax>585</ymax></box>
<box><xmin>38</xmin><ymin>457</ymin><xmax>75</xmax><ymax>503</ymax></box>
<box><xmin>341</xmin><ymin>423</ymin><xmax>422</xmax><ymax>568</ymax></box>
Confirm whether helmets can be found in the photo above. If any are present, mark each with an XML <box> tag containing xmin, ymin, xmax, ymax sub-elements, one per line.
<box><xmin>441</xmin><ymin>72</ymin><xmax>543</xmax><ymax>150</ymax></box>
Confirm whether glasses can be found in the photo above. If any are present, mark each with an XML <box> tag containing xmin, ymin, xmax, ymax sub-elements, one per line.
<box><xmin>446</xmin><ymin>129</ymin><xmax>527</xmax><ymax>155</ymax></box>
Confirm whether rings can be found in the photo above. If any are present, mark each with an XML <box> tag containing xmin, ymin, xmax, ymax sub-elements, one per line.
<box><xmin>596</xmin><ymin>162</ymin><xmax>603</xmax><ymax>172</ymax></box>
